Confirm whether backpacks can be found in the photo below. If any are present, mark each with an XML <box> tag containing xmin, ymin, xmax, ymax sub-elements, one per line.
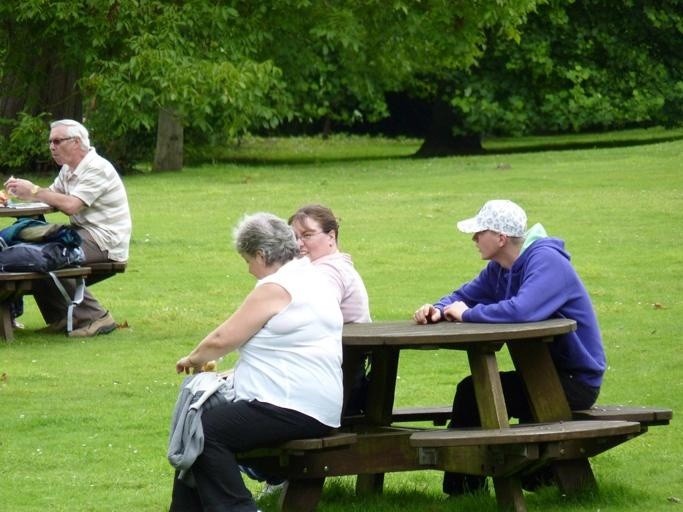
<box><xmin>0</xmin><ymin>245</ymin><xmax>86</xmax><ymax>331</ymax></box>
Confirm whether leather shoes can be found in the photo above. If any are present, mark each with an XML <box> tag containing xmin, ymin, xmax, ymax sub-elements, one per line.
<box><xmin>37</xmin><ymin>317</ymin><xmax>67</xmax><ymax>334</ymax></box>
<box><xmin>68</xmin><ymin>310</ymin><xmax>118</xmax><ymax>339</ymax></box>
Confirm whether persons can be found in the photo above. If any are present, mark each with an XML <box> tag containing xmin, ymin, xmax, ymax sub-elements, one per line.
<box><xmin>242</xmin><ymin>204</ymin><xmax>373</xmax><ymax>495</ymax></box>
<box><xmin>413</xmin><ymin>198</ymin><xmax>606</xmax><ymax>497</ymax></box>
<box><xmin>168</xmin><ymin>212</ymin><xmax>345</xmax><ymax>512</ymax></box>
<box><xmin>0</xmin><ymin>117</ymin><xmax>132</xmax><ymax>339</ymax></box>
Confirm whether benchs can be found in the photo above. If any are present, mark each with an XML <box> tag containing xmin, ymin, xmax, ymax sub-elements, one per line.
<box><xmin>0</xmin><ymin>266</ymin><xmax>92</xmax><ymax>281</ymax></box>
<box><xmin>274</xmin><ymin>404</ymin><xmax>673</xmax><ymax>476</ymax></box>
<box><xmin>85</xmin><ymin>261</ymin><xmax>126</xmax><ymax>286</ymax></box>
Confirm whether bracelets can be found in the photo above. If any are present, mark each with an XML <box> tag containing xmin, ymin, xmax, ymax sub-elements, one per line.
<box><xmin>188</xmin><ymin>358</ymin><xmax>198</xmax><ymax>367</ymax></box>
<box><xmin>31</xmin><ymin>185</ymin><xmax>41</xmax><ymax>198</ymax></box>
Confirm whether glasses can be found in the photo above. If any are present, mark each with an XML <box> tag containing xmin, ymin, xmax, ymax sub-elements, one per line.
<box><xmin>294</xmin><ymin>231</ymin><xmax>324</xmax><ymax>241</ymax></box>
<box><xmin>50</xmin><ymin>136</ymin><xmax>73</xmax><ymax>145</ymax></box>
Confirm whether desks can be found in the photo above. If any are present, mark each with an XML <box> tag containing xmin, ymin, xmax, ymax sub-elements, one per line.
<box><xmin>279</xmin><ymin>316</ymin><xmax>601</xmax><ymax>511</ymax></box>
<box><xmin>0</xmin><ymin>201</ymin><xmax>61</xmax><ymax>342</ymax></box>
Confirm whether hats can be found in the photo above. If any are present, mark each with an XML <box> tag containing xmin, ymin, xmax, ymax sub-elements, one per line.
<box><xmin>457</xmin><ymin>199</ymin><xmax>528</xmax><ymax>238</ymax></box>
